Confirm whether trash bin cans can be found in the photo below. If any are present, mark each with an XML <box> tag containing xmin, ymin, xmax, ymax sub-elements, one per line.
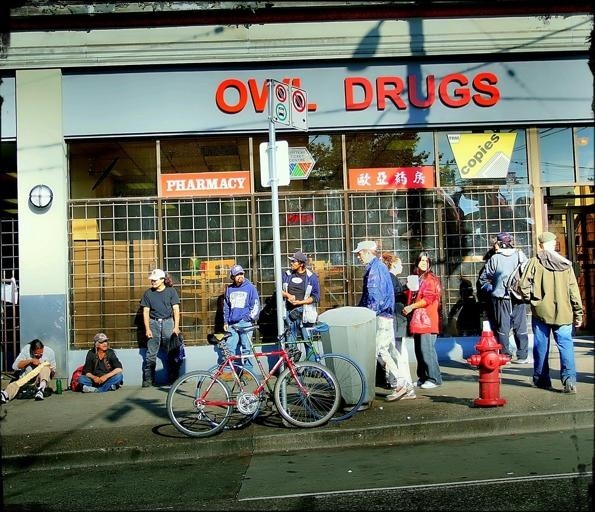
<box><xmin>317</xmin><ymin>306</ymin><xmax>377</xmax><ymax>411</ymax></box>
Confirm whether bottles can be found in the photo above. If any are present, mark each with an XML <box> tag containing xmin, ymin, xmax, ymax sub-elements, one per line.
<box><xmin>55</xmin><ymin>378</ymin><xmax>62</xmax><ymax>395</ymax></box>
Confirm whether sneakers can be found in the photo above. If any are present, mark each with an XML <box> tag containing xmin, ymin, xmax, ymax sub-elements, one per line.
<box><xmin>385</xmin><ymin>380</ymin><xmax>442</xmax><ymax>401</ymax></box>
<box><xmin>562</xmin><ymin>384</ymin><xmax>576</xmax><ymax>393</ymax></box>
<box><xmin>512</xmin><ymin>359</ymin><xmax>528</xmax><ymax>364</ymax></box>
<box><xmin>108</xmin><ymin>385</ymin><xmax>116</xmax><ymax>391</ymax></box>
<box><xmin>1</xmin><ymin>391</ymin><xmax>10</xmax><ymax>402</ymax></box>
<box><xmin>35</xmin><ymin>386</ymin><xmax>44</xmax><ymax>400</ymax></box>
<box><xmin>82</xmin><ymin>384</ymin><xmax>95</xmax><ymax>393</ymax></box>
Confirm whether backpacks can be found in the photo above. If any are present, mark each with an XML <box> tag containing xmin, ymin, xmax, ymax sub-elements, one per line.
<box><xmin>507</xmin><ymin>249</ymin><xmax>524</xmax><ymax>299</ymax></box>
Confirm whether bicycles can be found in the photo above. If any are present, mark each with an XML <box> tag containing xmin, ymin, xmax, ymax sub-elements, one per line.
<box><xmin>193</xmin><ymin>309</ymin><xmax>370</xmax><ymax>430</ymax></box>
<box><xmin>167</xmin><ymin>321</ymin><xmax>341</xmax><ymax>433</ymax></box>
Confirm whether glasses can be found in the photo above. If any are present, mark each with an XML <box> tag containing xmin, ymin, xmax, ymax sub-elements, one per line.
<box><xmin>33</xmin><ymin>352</ymin><xmax>44</xmax><ymax>356</ymax></box>
<box><xmin>293</xmin><ymin>259</ymin><xmax>298</xmax><ymax>263</ymax></box>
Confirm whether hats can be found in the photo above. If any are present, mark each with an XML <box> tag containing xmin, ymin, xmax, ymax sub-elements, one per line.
<box><xmin>94</xmin><ymin>333</ymin><xmax>110</xmax><ymax>342</ymax></box>
<box><xmin>230</xmin><ymin>264</ymin><xmax>245</xmax><ymax>276</ymax></box>
<box><xmin>288</xmin><ymin>251</ymin><xmax>307</xmax><ymax>262</ymax></box>
<box><xmin>148</xmin><ymin>269</ymin><xmax>166</xmax><ymax>280</ymax></box>
<box><xmin>350</xmin><ymin>240</ymin><xmax>378</xmax><ymax>253</ymax></box>
<box><xmin>537</xmin><ymin>231</ymin><xmax>556</xmax><ymax>243</ymax></box>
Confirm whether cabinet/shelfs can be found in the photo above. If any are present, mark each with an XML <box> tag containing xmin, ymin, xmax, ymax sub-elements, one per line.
<box><xmin>573</xmin><ymin>213</ymin><xmax>595</xmax><ymax>328</ymax></box>
<box><xmin>180</xmin><ymin>258</ymin><xmax>235</xmax><ymax>345</ymax></box>
<box><xmin>311</xmin><ymin>260</ymin><xmax>347</xmax><ymax>316</ymax></box>
<box><xmin>548</xmin><ymin>225</ymin><xmax>566</xmax><ymax>256</ymax></box>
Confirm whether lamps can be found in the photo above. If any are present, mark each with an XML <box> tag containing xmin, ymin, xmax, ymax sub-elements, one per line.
<box><xmin>29</xmin><ymin>184</ymin><xmax>53</xmax><ymax>208</ymax></box>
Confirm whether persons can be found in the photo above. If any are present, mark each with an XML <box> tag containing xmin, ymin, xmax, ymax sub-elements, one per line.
<box><xmin>381</xmin><ymin>250</ymin><xmax>413</xmax><ymax>382</ymax></box>
<box><xmin>491</xmin><ymin>238</ymin><xmax>502</xmax><ymax>254</ymax></box>
<box><xmin>478</xmin><ymin>231</ymin><xmax>531</xmax><ymax>366</ymax></box>
<box><xmin>139</xmin><ymin>268</ymin><xmax>186</xmax><ymax>388</ymax></box>
<box><xmin>402</xmin><ymin>251</ymin><xmax>444</xmax><ymax>390</ymax></box>
<box><xmin>211</xmin><ymin>264</ymin><xmax>262</xmax><ymax>383</ymax></box>
<box><xmin>77</xmin><ymin>331</ymin><xmax>125</xmax><ymax>393</ymax></box>
<box><xmin>350</xmin><ymin>240</ymin><xmax>417</xmax><ymax>403</ymax></box>
<box><xmin>280</xmin><ymin>250</ymin><xmax>321</xmax><ymax>379</ymax></box>
<box><xmin>518</xmin><ymin>231</ymin><xmax>586</xmax><ymax>394</ymax></box>
<box><xmin>0</xmin><ymin>337</ymin><xmax>58</xmax><ymax>405</ymax></box>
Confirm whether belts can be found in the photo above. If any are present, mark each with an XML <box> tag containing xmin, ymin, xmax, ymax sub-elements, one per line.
<box><xmin>149</xmin><ymin>314</ymin><xmax>171</xmax><ymax>321</ymax></box>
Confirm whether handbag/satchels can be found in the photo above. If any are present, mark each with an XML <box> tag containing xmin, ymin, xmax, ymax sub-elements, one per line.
<box><xmin>70</xmin><ymin>366</ymin><xmax>84</xmax><ymax>392</ymax></box>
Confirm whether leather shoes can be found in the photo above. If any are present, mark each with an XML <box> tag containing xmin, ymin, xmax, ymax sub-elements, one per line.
<box><xmin>214</xmin><ymin>372</ymin><xmax>235</xmax><ymax>383</ymax></box>
<box><xmin>241</xmin><ymin>370</ymin><xmax>253</xmax><ymax>380</ymax></box>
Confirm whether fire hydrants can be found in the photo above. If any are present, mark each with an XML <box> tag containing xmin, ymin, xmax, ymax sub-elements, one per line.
<box><xmin>469</xmin><ymin>330</ymin><xmax>513</xmax><ymax>411</ymax></box>
